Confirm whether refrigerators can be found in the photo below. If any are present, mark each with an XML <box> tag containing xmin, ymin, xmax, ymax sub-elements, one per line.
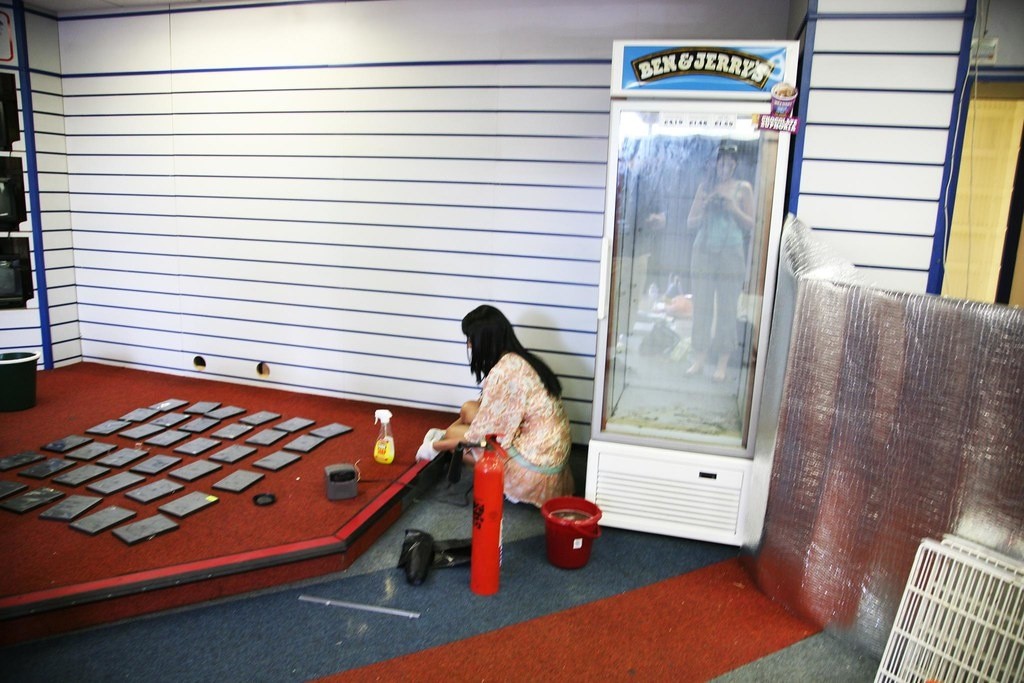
<box><xmin>583</xmin><ymin>38</ymin><xmax>802</xmax><ymax>550</ymax></box>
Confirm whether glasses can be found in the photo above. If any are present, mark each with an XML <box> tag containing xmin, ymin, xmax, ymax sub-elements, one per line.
<box><xmin>718</xmin><ymin>146</ymin><xmax>736</xmax><ymax>152</ymax></box>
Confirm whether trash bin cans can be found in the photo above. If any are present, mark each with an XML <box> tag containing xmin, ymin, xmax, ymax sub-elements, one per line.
<box><xmin>0</xmin><ymin>350</ymin><xmax>41</xmax><ymax>412</ymax></box>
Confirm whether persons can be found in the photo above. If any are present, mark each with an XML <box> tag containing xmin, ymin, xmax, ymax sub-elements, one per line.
<box><xmin>686</xmin><ymin>142</ymin><xmax>755</xmax><ymax>381</ymax></box>
<box><xmin>605</xmin><ymin>143</ymin><xmax>667</xmax><ymax>362</ymax></box>
<box><xmin>433</xmin><ymin>305</ymin><xmax>575</xmax><ymax>508</ymax></box>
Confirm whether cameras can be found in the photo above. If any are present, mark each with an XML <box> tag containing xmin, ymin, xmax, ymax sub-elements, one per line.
<box><xmin>711</xmin><ymin>191</ymin><xmax>727</xmax><ymax>213</ymax></box>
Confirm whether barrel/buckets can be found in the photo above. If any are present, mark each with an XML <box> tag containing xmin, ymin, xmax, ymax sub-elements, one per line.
<box><xmin>0</xmin><ymin>352</ymin><xmax>41</xmax><ymax>411</ymax></box>
<box><xmin>541</xmin><ymin>496</ymin><xmax>602</xmax><ymax>569</ymax></box>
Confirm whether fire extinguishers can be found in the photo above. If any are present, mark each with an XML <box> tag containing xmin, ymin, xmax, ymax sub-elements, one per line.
<box><xmin>448</xmin><ymin>433</ymin><xmax>509</xmax><ymax>596</ymax></box>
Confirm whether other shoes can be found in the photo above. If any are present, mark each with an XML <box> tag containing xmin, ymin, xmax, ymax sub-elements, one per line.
<box><xmin>683</xmin><ymin>364</ymin><xmax>702</xmax><ymax>375</ymax></box>
<box><xmin>712</xmin><ymin>370</ymin><xmax>725</xmax><ymax>383</ymax></box>
